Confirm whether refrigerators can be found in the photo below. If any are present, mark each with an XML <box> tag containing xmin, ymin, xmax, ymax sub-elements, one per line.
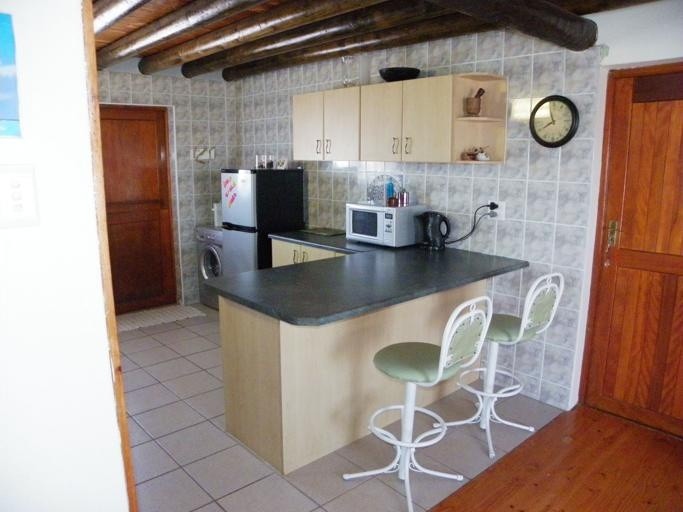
<box><xmin>219</xmin><ymin>167</ymin><xmax>303</xmax><ymax>276</ymax></box>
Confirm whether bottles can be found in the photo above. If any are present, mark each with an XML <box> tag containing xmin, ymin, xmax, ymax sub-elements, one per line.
<box><xmin>384</xmin><ymin>177</ymin><xmax>394</xmax><ymax>198</ymax></box>
<box><xmin>388</xmin><ymin>198</ymin><xmax>397</xmax><ymax>207</ymax></box>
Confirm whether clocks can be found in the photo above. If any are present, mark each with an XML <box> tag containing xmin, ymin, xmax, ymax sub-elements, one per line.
<box><xmin>528</xmin><ymin>94</ymin><xmax>579</xmax><ymax>149</ymax></box>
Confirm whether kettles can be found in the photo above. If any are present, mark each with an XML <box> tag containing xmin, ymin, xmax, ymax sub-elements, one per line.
<box><xmin>411</xmin><ymin>210</ymin><xmax>449</xmax><ymax>253</ymax></box>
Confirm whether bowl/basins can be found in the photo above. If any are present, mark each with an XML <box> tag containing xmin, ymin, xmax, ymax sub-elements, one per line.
<box><xmin>378</xmin><ymin>66</ymin><xmax>420</xmax><ymax>82</ymax></box>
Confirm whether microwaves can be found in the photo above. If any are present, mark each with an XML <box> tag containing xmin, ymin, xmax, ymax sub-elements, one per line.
<box><xmin>344</xmin><ymin>200</ymin><xmax>428</xmax><ymax>250</ymax></box>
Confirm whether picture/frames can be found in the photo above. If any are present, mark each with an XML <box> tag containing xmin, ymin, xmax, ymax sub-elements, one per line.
<box><xmin>276</xmin><ymin>157</ymin><xmax>287</xmax><ymax>170</ymax></box>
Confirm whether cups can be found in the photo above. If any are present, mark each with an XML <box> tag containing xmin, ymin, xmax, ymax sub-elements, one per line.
<box><xmin>463</xmin><ymin>96</ymin><xmax>482</xmax><ymax>118</ymax></box>
<box><xmin>254</xmin><ymin>153</ymin><xmax>289</xmax><ymax>171</ymax></box>
<box><xmin>398</xmin><ymin>192</ymin><xmax>409</xmax><ymax>204</ymax></box>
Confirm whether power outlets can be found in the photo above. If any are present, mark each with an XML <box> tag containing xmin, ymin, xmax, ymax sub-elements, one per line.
<box><xmin>488</xmin><ymin>200</ymin><xmax>505</xmax><ymax>221</ymax></box>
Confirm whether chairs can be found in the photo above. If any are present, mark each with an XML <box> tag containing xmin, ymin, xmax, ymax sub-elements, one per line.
<box><xmin>432</xmin><ymin>272</ymin><xmax>565</xmax><ymax>459</ymax></box>
<box><xmin>342</xmin><ymin>295</ymin><xmax>493</xmax><ymax>512</ymax></box>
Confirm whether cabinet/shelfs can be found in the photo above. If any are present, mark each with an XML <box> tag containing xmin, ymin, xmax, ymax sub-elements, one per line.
<box><xmin>291</xmin><ymin>86</ymin><xmax>360</xmax><ymax>162</ymax></box>
<box><xmin>360</xmin><ymin>72</ymin><xmax>508</xmax><ymax>164</ymax></box>
<box><xmin>270</xmin><ymin>239</ymin><xmax>336</xmax><ymax>267</ymax></box>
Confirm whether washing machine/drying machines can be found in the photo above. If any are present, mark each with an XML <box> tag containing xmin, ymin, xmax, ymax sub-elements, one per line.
<box><xmin>195</xmin><ymin>226</ymin><xmax>223</xmax><ymax>310</ymax></box>
